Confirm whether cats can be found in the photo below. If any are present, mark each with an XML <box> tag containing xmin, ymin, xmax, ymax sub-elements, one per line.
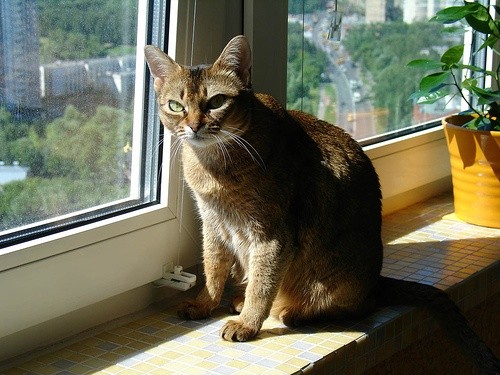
<box><xmin>143</xmin><ymin>35</ymin><xmax>500</xmax><ymax>375</ymax></box>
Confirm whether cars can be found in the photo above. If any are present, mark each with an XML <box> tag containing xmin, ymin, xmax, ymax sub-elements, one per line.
<box><xmin>288</xmin><ymin>1</ymin><xmax>381</xmax><ymax>139</ymax></box>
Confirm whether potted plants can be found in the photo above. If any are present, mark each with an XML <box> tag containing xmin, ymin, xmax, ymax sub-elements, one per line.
<box><xmin>405</xmin><ymin>0</ymin><xmax>500</xmax><ymax>231</ymax></box>
